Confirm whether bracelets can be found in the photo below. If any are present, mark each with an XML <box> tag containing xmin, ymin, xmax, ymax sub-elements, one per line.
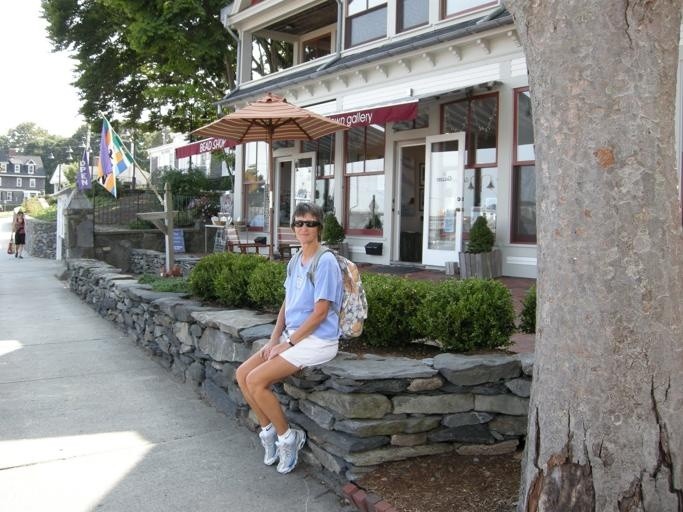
<box><xmin>286</xmin><ymin>337</ymin><xmax>293</xmax><ymax>346</ymax></box>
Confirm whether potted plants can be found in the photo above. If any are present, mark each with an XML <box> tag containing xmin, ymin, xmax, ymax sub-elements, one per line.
<box><xmin>457</xmin><ymin>215</ymin><xmax>503</xmax><ymax>280</ymax></box>
<box><xmin>320</xmin><ymin>211</ymin><xmax>350</xmax><ymax>261</ymax></box>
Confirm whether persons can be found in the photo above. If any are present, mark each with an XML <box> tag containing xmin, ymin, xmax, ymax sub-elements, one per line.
<box><xmin>7</xmin><ymin>211</ymin><xmax>25</xmax><ymax>258</ymax></box>
<box><xmin>232</xmin><ymin>202</ymin><xmax>367</xmax><ymax>474</ymax></box>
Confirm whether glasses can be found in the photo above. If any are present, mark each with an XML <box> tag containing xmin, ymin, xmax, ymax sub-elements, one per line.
<box><xmin>292</xmin><ymin>220</ymin><xmax>320</xmax><ymax>228</ymax></box>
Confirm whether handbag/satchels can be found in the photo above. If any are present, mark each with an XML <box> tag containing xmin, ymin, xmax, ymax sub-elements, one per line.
<box><xmin>7</xmin><ymin>239</ymin><xmax>16</xmax><ymax>254</ymax></box>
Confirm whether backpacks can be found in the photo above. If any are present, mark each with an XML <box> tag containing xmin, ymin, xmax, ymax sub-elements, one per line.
<box><xmin>289</xmin><ymin>245</ymin><xmax>369</xmax><ymax>340</ymax></box>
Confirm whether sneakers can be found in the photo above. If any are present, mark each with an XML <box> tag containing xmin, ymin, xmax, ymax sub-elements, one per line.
<box><xmin>259</xmin><ymin>423</ymin><xmax>279</xmax><ymax>466</ymax></box>
<box><xmin>276</xmin><ymin>428</ymin><xmax>307</xmax><ymax>474</ymax></box>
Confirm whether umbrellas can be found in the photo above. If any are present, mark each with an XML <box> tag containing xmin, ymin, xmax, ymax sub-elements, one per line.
<box><xmin>188</xmin><ymin>92</ymin><xmax>349</xmax><ymax>258</ymax></box>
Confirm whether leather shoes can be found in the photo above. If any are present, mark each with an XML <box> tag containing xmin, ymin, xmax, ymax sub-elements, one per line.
<box><xmin>15</xmin><ymin>252</ymin><xmax>24</xmax><ymax>259</ymax></box>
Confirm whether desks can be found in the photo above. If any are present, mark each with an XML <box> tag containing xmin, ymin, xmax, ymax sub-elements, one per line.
<box><xmin>204</xmin><ymin>224</ymin><xmax>274</xmax><ymax>258</ymax></box>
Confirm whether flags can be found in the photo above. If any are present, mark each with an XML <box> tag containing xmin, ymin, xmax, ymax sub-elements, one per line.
<box><xmin>95</xmin><ymin>117</ymin><xmax>135</xmax><ymax>199</ymax></box>
<box><xmin>74</xmin><ymin>148</ymin><xmax>91</xmax><ymax>192</ymax></box>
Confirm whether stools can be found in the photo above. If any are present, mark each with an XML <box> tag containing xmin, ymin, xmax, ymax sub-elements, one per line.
<box><xmin>280</xmin><ymin>246</ymin><xmax>300</xmax><ymax>262</ymax></box>
